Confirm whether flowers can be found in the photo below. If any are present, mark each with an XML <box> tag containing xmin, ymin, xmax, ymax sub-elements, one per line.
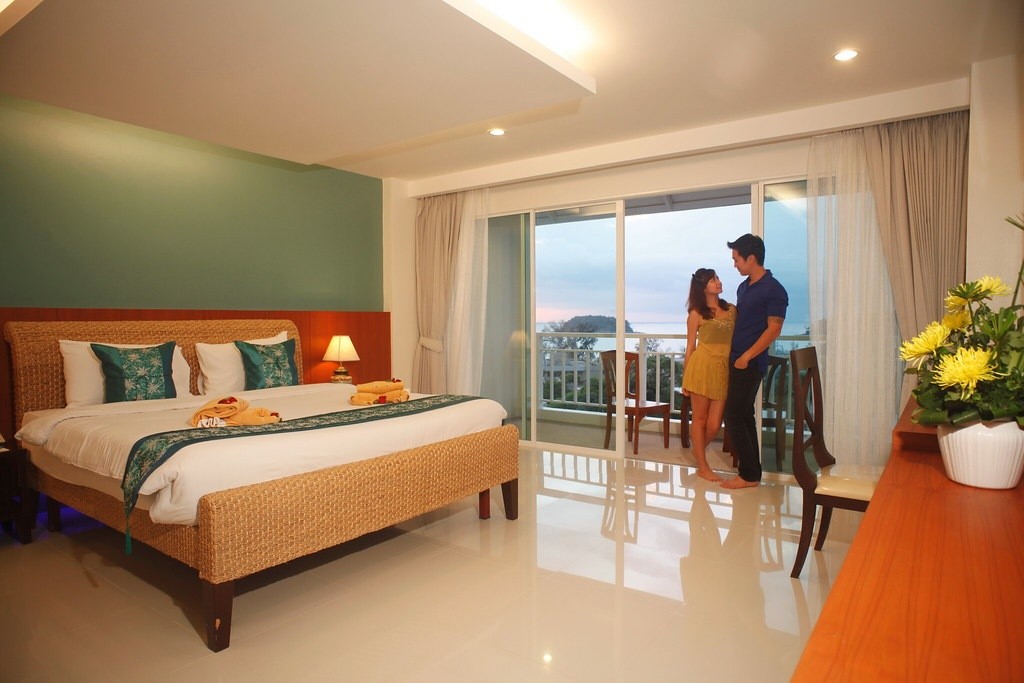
<box><xmin>898</xmin><ymin>212</ymin><xmax>1024</xmax><ymax>430</ymax></box>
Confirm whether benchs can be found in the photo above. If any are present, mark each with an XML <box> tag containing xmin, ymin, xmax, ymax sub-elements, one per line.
<box><xmin>674</xmin><ymin>386</ymin><xmax>691</xmax><ymax>448</ymax></box>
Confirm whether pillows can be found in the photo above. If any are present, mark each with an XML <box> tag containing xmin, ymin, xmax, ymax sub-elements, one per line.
<box><xmin>233</xmin><ymin>338</ymin><xmax>299</xmax><ymax>391</ymax></box>
<box><xmin>195</xmin><ymin>330</ymin><xmax>289</xmax><ymax>396</ymax></box>
<box><xmin>90</xmin><ymin>340</ymin><xmax>177</xmax><ymax>404</ymax></box>
<box><xmin>58</xmin><ymin>339</ymin><xmax>191</xmax><ymax>407</ymax></box>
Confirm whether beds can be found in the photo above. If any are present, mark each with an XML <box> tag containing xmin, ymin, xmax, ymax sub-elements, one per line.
<box><xmin>2</xmin><ymin>319</ymin><xmax>520</xmax><ymax>652</ymax></box>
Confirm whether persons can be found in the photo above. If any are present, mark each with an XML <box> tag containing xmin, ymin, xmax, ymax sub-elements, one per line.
<box><xmin>682</xmin><ymin>268</ymin><xmax>736</xmax><ymax>482</ymax></box>
<box><xmin>719</xmin><ymin>233</ymin><xmax>789</xmax><ymax>489</ymax></box>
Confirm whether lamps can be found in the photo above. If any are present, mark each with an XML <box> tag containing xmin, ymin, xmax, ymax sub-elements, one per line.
<box><xmin>321</xmin><ymin>334</ymin><xmax>361</xmax><ymax>384</ymax></box>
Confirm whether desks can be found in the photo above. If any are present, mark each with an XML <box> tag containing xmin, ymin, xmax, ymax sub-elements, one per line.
<box><xmin>1</xmin><ymin>439</ymin><xmax>32</xmax><ymax>545</ymax></box>
<box><xmin>791</xmin><ymin>397</ymin><xmax>1023</xmax><ymax>683</ymax></box>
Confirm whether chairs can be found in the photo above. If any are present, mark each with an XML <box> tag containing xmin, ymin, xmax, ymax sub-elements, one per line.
<box><xmin>600</xmin><ymin>351</ymin><xmax>671</xmax><ymax>455</ymax></box>
<box><xmin>789</xmin><ymin>346</ymin><xmax>887</xmax><ymax>581</ymax></box>
<box><xmin>722</xmin><ymin>354</ymin><xmax>787</xmax><ymax>471</ymax></box>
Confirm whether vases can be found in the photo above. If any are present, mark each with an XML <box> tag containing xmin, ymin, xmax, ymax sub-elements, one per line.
<box><xmin>935</xmin><ymin>421</ymin><xmax>1024</xmax><ymax>489</ymax></box>
